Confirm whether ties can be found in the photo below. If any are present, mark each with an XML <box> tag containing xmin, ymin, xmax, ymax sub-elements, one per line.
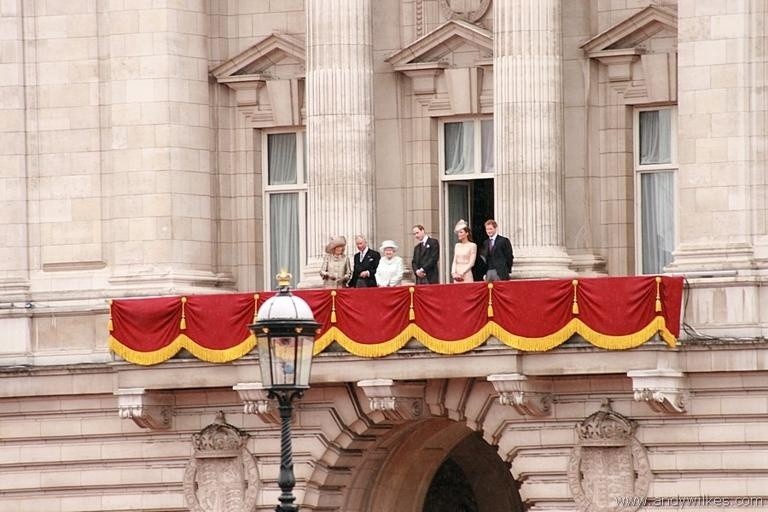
<box><xmin>420</xmin><ymin>242</ymin><xmax>423</xmax><ymax>252</ymax></box>
<box><xmin>360</xmin><ymin>251</ymin><xmax>363</xmax><ymax>263</ymax></box>
<box><xmin>489</xmin><ymin>239</ymin><xmax>493</xmax><ymax>254</ymax></box>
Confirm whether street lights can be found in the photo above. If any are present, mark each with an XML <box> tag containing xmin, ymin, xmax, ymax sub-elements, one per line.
<box><xmin>246</xmin><ymin>266</ymin><xmax>326</xmax><ymax>512</ymax></box>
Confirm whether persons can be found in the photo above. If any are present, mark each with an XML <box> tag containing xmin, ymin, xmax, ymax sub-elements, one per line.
<box><xmin>375</xmin><ymin>239</ymin><xmax>403</xmax><ymax>286</ymax></box>
<box><xmin>319</xmin><ymin>236</ymin><xmax>351</xmax><ymax>287</ymax></box>
<box><xmin>451</xmin><ymin>218</ymin><xmax>477</xmax><ymax>282</ymax></box>
<box><xmin>348</xmin><ymin>233</ymin><xmax>380</xmax><ymax>288</ymax></box>
<box><xmin>480</xmin><ymin>219</ymin><xmax>514</xmax><ymax>281</ymax></box>
<box><xmin>411</xmin><ymin>224</ymin><xmax>440</xmax><ymax>284</ymax></box>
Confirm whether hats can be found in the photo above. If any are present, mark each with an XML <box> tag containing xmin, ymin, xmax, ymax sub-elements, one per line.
<box><xmin>326</xmin><ymin>235</ymin><xmax>346</xmax><ymax>252</ymax></box>
<box><xmin>454</xmin><ymin>219</ymin><xmax>468</xmax><ymax>233</ymax></box>
<box><xmin>380</xmin><ymin>240</ymin><xmax>399</xmax><ymax>254</ymax></box>
